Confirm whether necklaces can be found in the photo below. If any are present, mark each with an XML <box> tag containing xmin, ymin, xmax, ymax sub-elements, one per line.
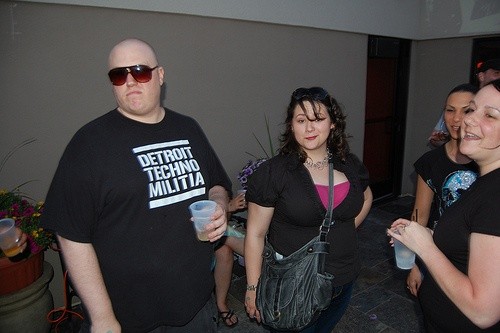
<box><xmin>304</xmin><ymin>147</ymin><xmax>333</xmax><ymax>170</ymax></box>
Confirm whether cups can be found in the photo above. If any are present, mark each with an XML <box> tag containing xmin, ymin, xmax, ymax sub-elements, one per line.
<box><xmin>189</xmin><ymin>200</ymin><xmax>217</xmax><ymax>241</ymax></box>
<box><xmin>0</xmin><ymin>218</ymin><xmax>21</xmax><ymax>257</ymax></box>
<box><xmin>392</xmin><ymin>237</ymin><xmax>415</xmax><ymax>269</ymax></box>
<box><xmin>237</xmin><ymin>190</ymin><xmax>247</xmax><ymax>206</ymax></box>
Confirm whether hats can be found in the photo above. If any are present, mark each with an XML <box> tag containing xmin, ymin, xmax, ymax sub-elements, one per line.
<box><xmin>474</xmin><ymin>59</ymin><xmax>500</xmax><ymax>73</ymax></box>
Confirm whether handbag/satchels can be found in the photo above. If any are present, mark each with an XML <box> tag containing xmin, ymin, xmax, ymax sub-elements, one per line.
<box><xmin>254</xmin><ymin>219</ymin><xmax>336</xmax><ymax>332</ymax></box>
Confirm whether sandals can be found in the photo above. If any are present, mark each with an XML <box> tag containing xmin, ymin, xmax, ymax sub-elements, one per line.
<box><xmin>219</xmin><ymin>308</ymin><xmax>238</xmax><ymax>328</ymax></box>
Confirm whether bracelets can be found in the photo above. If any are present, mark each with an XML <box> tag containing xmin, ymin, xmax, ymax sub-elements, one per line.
<box><xmin>246</xmin><ymin>284</ymin><xmax>257</xmax><ymax>291</ymax></box>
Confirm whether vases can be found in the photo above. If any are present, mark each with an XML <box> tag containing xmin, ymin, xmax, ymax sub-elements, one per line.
<box><xmin>0</xmin><ymin>246</ymin><xmax>44</xmax><ymax>296</ymax></box>
<box><xmin>0</xmin><ymin>260</ymin><xmax>54</xmax><ymax>333</ymax></box>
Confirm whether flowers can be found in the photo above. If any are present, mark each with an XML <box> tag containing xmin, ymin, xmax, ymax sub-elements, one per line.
<box><xmin>236</xmin><ymin>111</ymin><xmax>281</xmax><ymax>191</ymax></box>
<box><xmin>0</xmin><ymin>136</ymin><xmax>57</xmax><ymax>256</ymax></box>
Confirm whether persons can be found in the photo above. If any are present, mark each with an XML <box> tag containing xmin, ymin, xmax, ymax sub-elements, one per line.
<box><xmin>387</xmin><ymin>54</ymin><xmax>500</xmax><ymax>333</ymax></box>
<box><xmin>245</xmin><ymin>88</ymin><xmax>373</xmax><ymax>333</ymax></box>
<box><xmin>214</xmin><ymin>193</ymin><xmax>245</xmax><ymax>328</ymax></box>
<box><xmin>38</xmin><ymin>39</ymin><xmax>233</xmax><ymax>333</ymax></box>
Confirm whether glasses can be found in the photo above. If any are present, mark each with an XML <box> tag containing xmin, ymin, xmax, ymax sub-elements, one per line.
<box><xmin>291</xmin><ymin>87</ymin><xmax>329</xmax><ymax>101</ymax></box>
<box><xmin>108</xmin><ymin>65</ymin><xmax>159</xmax><ymax>87</ymax></box>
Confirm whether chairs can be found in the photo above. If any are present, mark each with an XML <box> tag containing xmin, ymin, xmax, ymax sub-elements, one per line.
<box><xmin>55</xmin><ymin>240</ymin><xmax>92</xmax><ymax>328</ymax></box>
